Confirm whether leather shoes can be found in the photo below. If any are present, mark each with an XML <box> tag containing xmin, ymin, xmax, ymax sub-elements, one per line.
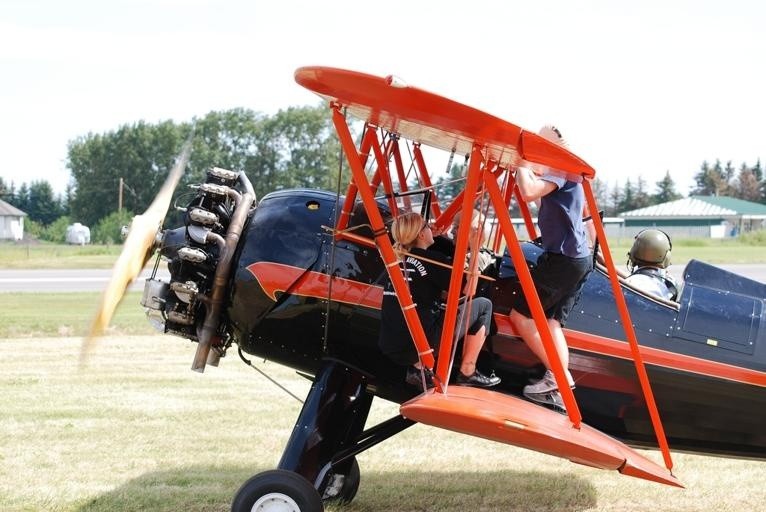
<box><xmin>404</xmin><ymin>364</ymin><xmax>435</xmax><ymax>392</ymax></box>
<box><xmin>454</xmin><ymin>368</ymin><xmax>502</xmax><ymax>388</ymax></box>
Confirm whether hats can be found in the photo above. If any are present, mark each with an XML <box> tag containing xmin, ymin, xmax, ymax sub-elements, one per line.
<box><xmin>537</xmin><ymin>123</ymin><xmax>568</xmax><ymax>148</ymax></box>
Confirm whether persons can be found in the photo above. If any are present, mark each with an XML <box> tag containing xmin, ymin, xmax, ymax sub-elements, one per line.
<box><xmin>515</xmin><ymin>123</ymin><xmax>594</xmax><ymax>415</ymax></box>
<box><xmin>624</xmin><ymin>228</ymin><xmax>681</xmax><ymax>301</ymax></box>
<box><xmin>381</xmin><ymin>212</ymin><xmax>502</xmax><ymax>389</ymax></box>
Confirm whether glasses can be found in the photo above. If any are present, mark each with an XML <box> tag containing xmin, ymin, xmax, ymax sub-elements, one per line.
<box><xmin>420</xmin><ymin>223</ymin><xmax>429</xmax><ymax>231</ymax></box>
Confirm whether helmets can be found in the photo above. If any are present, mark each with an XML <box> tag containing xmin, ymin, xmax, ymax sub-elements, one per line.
<box><xmin>629</xmin><ymin>227</ymin><xmax>673</xmax><ymax>269</ymax></box>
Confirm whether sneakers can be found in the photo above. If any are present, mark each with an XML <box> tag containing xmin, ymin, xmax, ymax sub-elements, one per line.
<box><xmin>523</xmin><ymin>369</ymin><xmax>577</xmax><ymax>395</ymax></box>
<box><xmin>523</xmin><ymin>388</ymin><xmax>569</xmax><ymax>416</ymax></box>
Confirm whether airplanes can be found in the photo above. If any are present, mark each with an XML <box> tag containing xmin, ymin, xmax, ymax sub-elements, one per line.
<box><xmin>72</xmin><ymin>63</ymin><xmax>766</xmax><ymax>512</ymax></box>
